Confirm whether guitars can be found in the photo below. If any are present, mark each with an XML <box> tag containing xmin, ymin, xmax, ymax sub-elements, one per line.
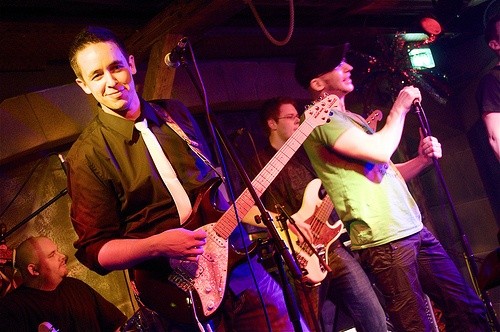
<box><xmin>38</xmin><ymin>322</ymin><xmax>59</xmax><ymax>332</ymax></box>
<box><xmin>275</xmin><ymin>109</ymin><xmax>383</xmax><ymax>288</ymax></box>
<box><xmin>127</xmin><ymin>92</ymin><xmax>341</xmax><ymax>320</ymax></box>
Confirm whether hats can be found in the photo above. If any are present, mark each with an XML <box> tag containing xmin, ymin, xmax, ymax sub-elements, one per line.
<box><xmin>295</xmin><ymin>44</ymin><xmax>350</xmax><ymax>85</ymax></box>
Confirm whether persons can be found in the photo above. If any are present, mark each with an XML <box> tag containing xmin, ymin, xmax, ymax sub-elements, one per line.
<box><xmin>252</xmin><ymin>40</ymin><xmax>487</xmax><ymax>332</ymax></box>
<box><xmin>476</xmin><ymin>0</ymin><xmax>500</xmax><ymax>164</ymax></box>
<box><xmin>0</xmin><ymin>237</ymin><xmax>127</xmax><ymax>332</ymax></box>
<box><xmin>65</xmin><ymin>27</ymin><xmax>314</xmax><ymax>332</ymax></box>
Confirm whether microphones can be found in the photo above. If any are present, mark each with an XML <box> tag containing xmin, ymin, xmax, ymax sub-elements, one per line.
<box><xmin>414</xmin><ymin>97</ymin><xmax>421</xmax><ymax>108</ymax></box>
<box><xmin>164</xmin><ymin>38</ymin><xmax>187</xmax><ymax>67</ymax></box>
<box><xmin>227</xmin><ymin>128</ymin><xmax>246</xmax><ymax>140</ymax></box>
<box><xmin>58</xmin><ymin>155</ymin><xmax>68</xmax><ymax>176</ymax></box>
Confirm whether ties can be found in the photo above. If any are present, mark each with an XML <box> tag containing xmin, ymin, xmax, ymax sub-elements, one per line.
<box><xmin>134</xmin><ymin>119</ymin><xmax>194</xmax><ymax>226</ymax></box>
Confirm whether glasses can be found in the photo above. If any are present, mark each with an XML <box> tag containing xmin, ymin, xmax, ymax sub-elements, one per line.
<box><xmin>278</xmin><ymin>114</ymin><xmax>300</xmax><ymax>121</ymax></box>
<box><xmin>333</xmin><ymin>59</ymin><xmax>346</xmax><ymax>70</ymax></box>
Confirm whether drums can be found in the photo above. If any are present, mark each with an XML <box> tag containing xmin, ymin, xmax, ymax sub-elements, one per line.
<box><xmin>114</xmin><ymin>306</ymin><xmax>165</xmax><ymax>332</ymax></box>
